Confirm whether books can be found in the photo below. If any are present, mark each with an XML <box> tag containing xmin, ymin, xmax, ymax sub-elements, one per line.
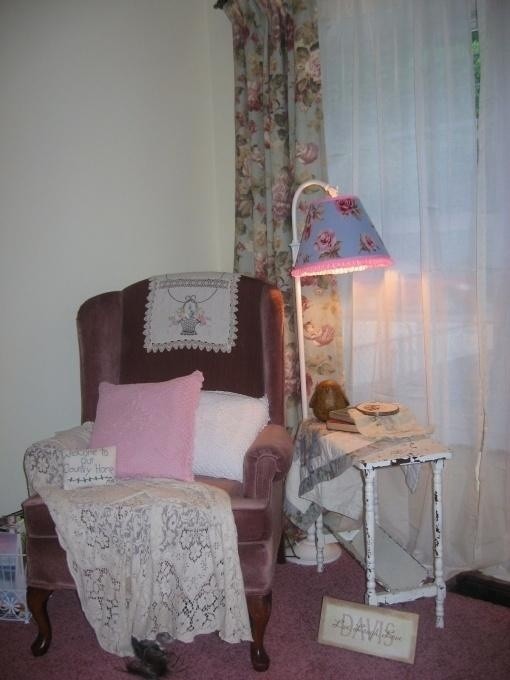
<box><xmin>326</xmin><ymin>406</ymin><xmax>361</xmax><ymax>432</ymax></box>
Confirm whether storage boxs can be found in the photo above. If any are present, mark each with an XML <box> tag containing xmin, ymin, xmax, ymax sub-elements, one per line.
<box><xmin>0</xmin><ymin>533</ymin><xmax>32</xmax><ymax>623</ymax></box>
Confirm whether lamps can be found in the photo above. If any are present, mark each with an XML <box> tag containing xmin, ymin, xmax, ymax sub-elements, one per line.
<box><xmin>285</xmin><ymin>179</ymin><xmax>393</xmax><ymax>567</ymax></box>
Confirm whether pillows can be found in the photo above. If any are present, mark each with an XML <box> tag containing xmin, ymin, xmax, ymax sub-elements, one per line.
<box><xmin>92</xmin><ymin>371</ymin><xmax>202</xmax><ymax>483</ymax></box>
<box><xmin>63</xmin><ymin>448</ymin><xmax>117</xmax><ymax>489</ymax></box>
<box><xmin>193</xmin><ymin>389</ymin><xmax>270</xmax><ymax>482</ymax></box>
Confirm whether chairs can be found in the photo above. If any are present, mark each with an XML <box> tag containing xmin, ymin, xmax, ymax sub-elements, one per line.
<box><xmin>21</xmin><ymin>272</ymin><xmax>294</xmax><ymax>672</ymax></box>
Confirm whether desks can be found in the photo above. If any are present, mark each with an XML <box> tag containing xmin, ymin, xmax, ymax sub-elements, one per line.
<box><xmin>303</xmin><ymin>420</ymin><xmax>453</xmax><ymax>630</ymax></box>
